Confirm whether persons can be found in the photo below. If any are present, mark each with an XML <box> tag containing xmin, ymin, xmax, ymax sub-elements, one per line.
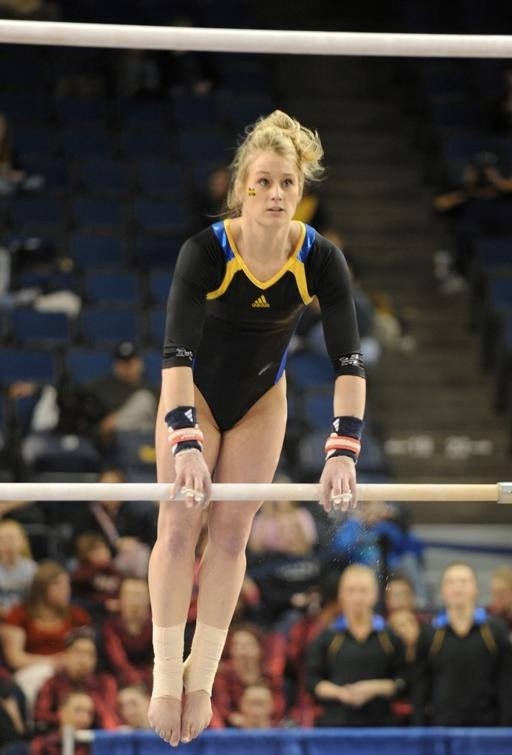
<box><xmin>1</xmin><ymin>471</ymin><xmax>510</xmax><ymax>755</ymax></box>
<box><xmin>292</xmin><ymin>153</ymin><xmax>511</xmax><ymax>355</ymax></box>
<box><xmin>145</xmin><ymin>111</ymin><xmax>367</xmax><ymax>749</ymax></box>
<box><xmin>0</xmin><ymin>339</ymin><xmax>161</xmax><ymax>478</ymax></box>
<box><xmin>1</xmin><ymin>158</ymin><xmax>89</xmax><ymax>320</ymax></box>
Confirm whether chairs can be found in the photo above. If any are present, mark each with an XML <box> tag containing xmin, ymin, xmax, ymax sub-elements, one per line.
<box><xmin>401</xmin><ymin>54</ymin><xmax>511</xmax><ymax>419</ymax></box>
<box><xmin>0</xmin><ymin>43</ymin><xmax>395</xmax><ymax>496</ymax></box>
